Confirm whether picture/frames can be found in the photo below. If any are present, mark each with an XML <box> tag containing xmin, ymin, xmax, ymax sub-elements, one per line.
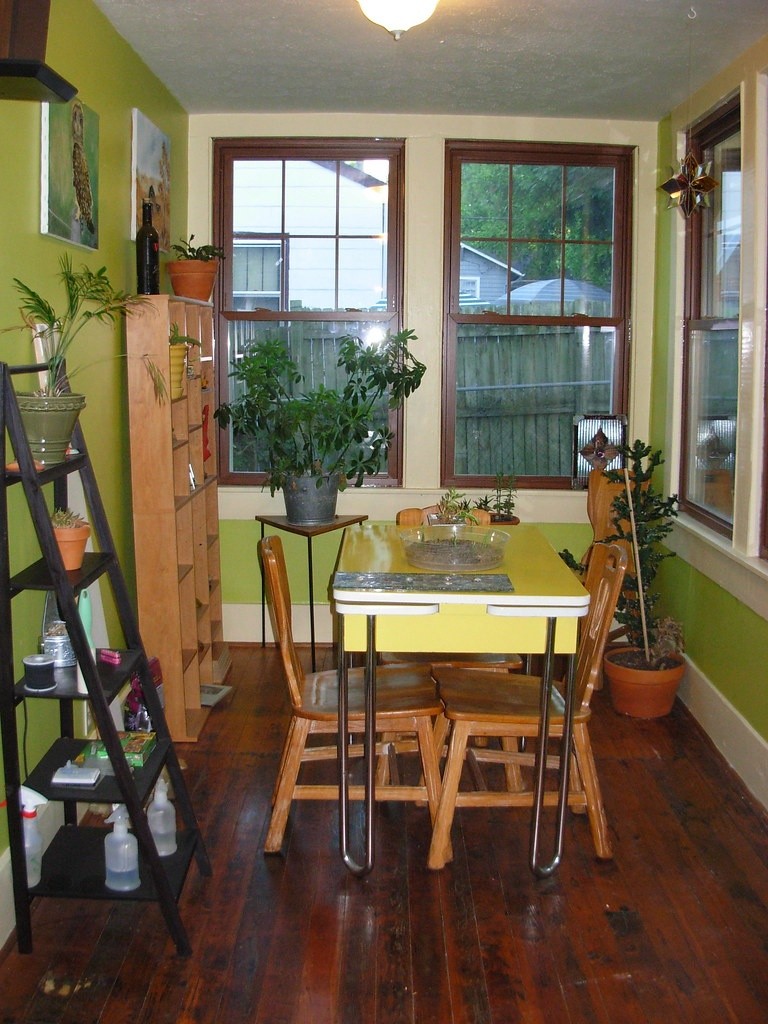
<box><xmin>570</xmin><ymin>413</ymin><xmax>628</xmax><ymax>491</ymax></box>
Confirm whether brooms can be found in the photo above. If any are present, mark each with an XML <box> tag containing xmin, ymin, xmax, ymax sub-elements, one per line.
<box><xmin>78</xmin><ymin>590</ymin><xmax>115</xmax><ymax>829</ymax></box>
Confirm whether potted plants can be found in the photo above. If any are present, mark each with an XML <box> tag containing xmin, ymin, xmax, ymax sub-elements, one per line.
<box><xmin>49</xmin><ymin>503</ymin><xmax>91</xmax><ymax>572</ymax></box>
<box><xmin>215</xmin><ymin>326</ymin><xmax>428</xmax><ymax>529</ymax></box>
<box><xmin>438</xmin><ymin>467</ymin><xmax>521</xmax><ymax>524</ymax></box>
<box><xmin>167</xmin><ymin>233</ymin><xmax>225</xmax><ymax>302</ymax></box>
<box><xmin>10</xmin><ymin>253</ymin><xmax>169</xmax><ymax>471</ymax></box>
<box><xmin>597</xmin><ymin>436</ymin><xmax>687</xmax><ymax>719</ymax></box>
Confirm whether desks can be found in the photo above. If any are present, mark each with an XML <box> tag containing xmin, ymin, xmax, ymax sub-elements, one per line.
<box><xmin>257</xmin><ymin>515</ymin><xmax>369</xmax><ymax>671</ymax></box>
<box><xmin>329</xmin><ymin>522</ymin><xmax>594</xmax><ymax>877</ymax></box>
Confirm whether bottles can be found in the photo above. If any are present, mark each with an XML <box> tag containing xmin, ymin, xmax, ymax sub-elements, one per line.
<box><xmin>135</xmin><ymin>198</ymin><xmax>160</xmax><ymax>295</ymax></box>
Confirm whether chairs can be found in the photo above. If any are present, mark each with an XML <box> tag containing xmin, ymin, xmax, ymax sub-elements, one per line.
<box><xmin>362</xmin><ymin>503</ymin><xmax>524</xmax><ymax>811</ymax></box>
<box><xmin>260</xmin><ymin>534</ymin><xmax>456</xmax><ymax>865</ymax></box>
<box><xmin>427</xmin><ymin>542</ymin><xmax>628</xmax><ymax>871</ymax></box>
<box><xmin>579</xmin><ymin>468</ymin><xmax>651</xmax><ymax>650</ymax></box>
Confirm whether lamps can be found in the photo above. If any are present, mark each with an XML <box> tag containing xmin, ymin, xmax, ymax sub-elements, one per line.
<box><xmin>357</xmin><ymin>0</ymin><xmax>438</xmax><ymax>41</ymax></box>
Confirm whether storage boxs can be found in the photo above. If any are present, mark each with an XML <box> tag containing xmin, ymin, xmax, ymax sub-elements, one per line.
<box><xmin>96</xmin><ymin>732</ymin><xmax>157</xmax><ymax>767</ymax></box>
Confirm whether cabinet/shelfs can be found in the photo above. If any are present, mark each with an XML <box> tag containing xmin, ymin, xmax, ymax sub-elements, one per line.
<box><xmin>124</xmin><ymin>294</ymin><xmax>235</xmax><ymax>744</ymax></box>
<box><xmin>0</xmin><ymin>357</ymin><xmax>212</xmax><ymax>960</ymax></box>
